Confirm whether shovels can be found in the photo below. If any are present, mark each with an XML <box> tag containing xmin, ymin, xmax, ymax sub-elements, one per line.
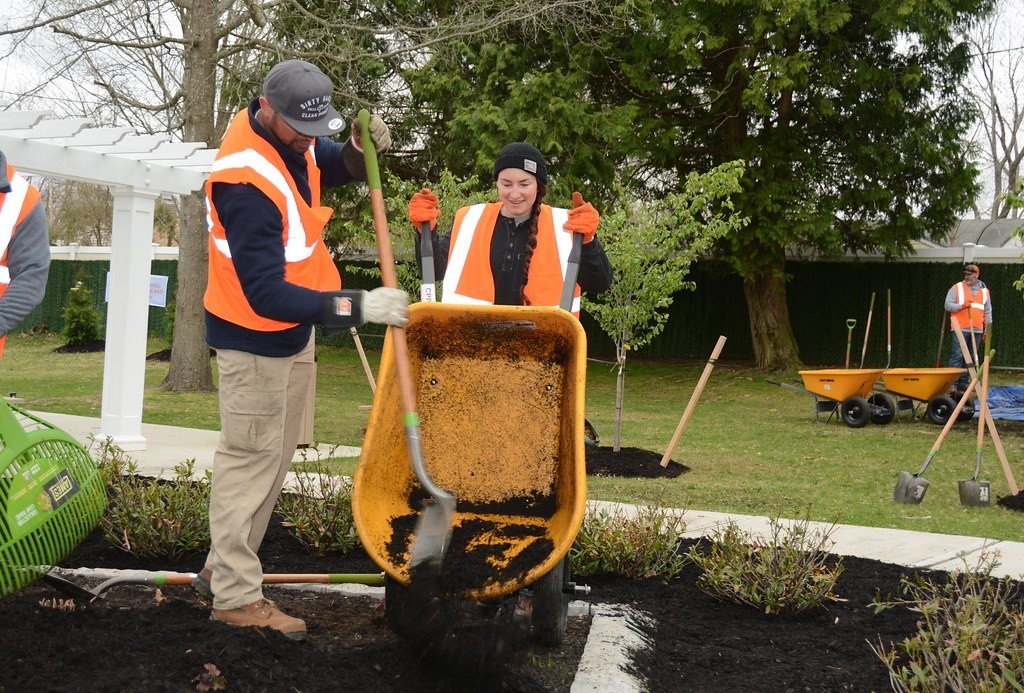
<box><xmin>893</xmin><ymin>348</ymin><xmax>999</xmax><ymax>503</ymax></box>
<box><xmin>358</xmin><ymin>111</ymin><xmax>455</xmax><ymax>588</ymax></box>
<box><xmin>44</xmin><ymin>565</ymin><xmax>386</xmax><ymax>606</ymax></box>
<box><xmin>958</xmin><ymin>323</ymin><xmax>992</xmax><ymax>507</ymax></box>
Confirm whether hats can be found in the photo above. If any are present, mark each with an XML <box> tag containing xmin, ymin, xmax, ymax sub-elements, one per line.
<box><xmin>494</xmin><ymin>142</ymin><xmax>547</xmax><ymax>186</ymax></box>
<box><xmin>962</xmin><ymin>265</ymin><xmax>979</xmax><ymax>275</ymax></box>
<box><xmin>263</xmin><ymin>61</ymin><xmax>346</xmax><ymax>137</ymax></box>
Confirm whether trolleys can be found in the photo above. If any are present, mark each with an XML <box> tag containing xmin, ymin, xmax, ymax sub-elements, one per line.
<box><xmin>344</xmin><ymin>221</ymin><xmax>590</xmax><ymax>658</ymax></box>
<box><xmin>871</xmin><ymin>366</ymin><xmax>976</xmax><ymax>425</ymax></box>
<box><xmin>765</xmin><ymin>368</ymin><xmax>895</xmax><ymax>428</ymax></box>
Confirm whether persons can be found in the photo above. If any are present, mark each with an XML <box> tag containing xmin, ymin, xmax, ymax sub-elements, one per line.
<box><xmin>944</xmin><ymin>265</ymin><xmax>992</xmax><ymax>394</ymax></box>
<box><xmin>408</xmin><ymin>141</ymin><xmax>614</xmax><ymax>320</ymax></box>
<box><xmin>0</xmin><ymin>151</ymin><xmax>50</xmax><ymax>363</ymax></box>
<box><xmin>203</xmin><ymin>60</ymin><xmax>411</xmax><ymax>641</ymax></box>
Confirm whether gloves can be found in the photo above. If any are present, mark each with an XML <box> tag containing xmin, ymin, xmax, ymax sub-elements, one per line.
<box><xmin>351</xmin><ymin>116</ymin><xmax>392</xmax><ymax>154</ymax></box>
<box><xmin>962</xmin><ymin>300</ymin><xmax>973</xmax><ymax>310</ymax></box>
<box><xmin>982</xmin><ymin>332</ymin><xmax>986</xmax><ymax>342</ymax></box>
<box><xmin>563</xmin><ymin>192</ymin><xmax>599</xmax><ymax>244</ymax></box>
<box><xmin>323</xmin><ymin>287</ymin><xmax>409</xmax><ymax>336</ymax></box>
<box><xmin>409</xmin><ymin>189</ymin><xmax>443</xmax><ymax>234</ymax></box>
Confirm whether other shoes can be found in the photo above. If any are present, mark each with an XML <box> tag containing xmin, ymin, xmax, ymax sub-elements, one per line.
<box><xmin>958</xmin><ymin>385</ymin><xmax>975</xmax><ymax>392</ymax></box>
<box><xmin>948</xmin><ymin>385</ymin><xmax>957</xmax><ymax>393</ymax></box>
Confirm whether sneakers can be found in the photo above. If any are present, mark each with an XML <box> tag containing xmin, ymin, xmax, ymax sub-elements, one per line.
<box><xmin>190</xmin><ymin>568</ymin><xmax>213</xmax><ymax>601</ymax></box>
<box><xmin>210</xmin><ymin>598</ymin><xmax>306</xmax><ymax>641</ymax></box>
